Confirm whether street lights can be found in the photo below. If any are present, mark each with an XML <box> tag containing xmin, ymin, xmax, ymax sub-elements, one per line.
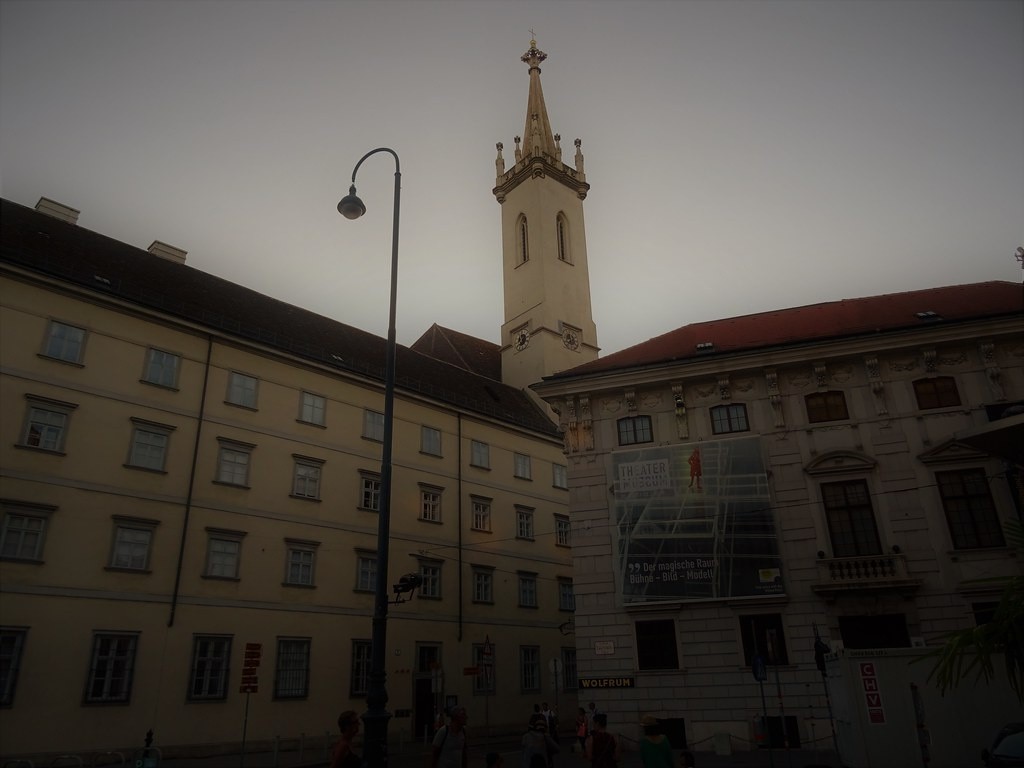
<box><xmin>336</xmin><ymin>149</ymin><xmax>422</xmax><ymax>768</ymax></box>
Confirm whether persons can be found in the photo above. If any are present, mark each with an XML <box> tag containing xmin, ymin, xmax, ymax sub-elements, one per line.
<box><xmin>574</xmin><ymin>709</ymin><xmax>588</xmax><ymax>757</ymax></box>
<box><xmin>485</xmin><ymin>752</ymin><xmax>501</xmax><ymax>768</ymax></box>
<box><xmin>520</xmin><ymin>703</ymin><xmax>560</xmax><ymax>768</ymax></box>
<box><xmin>589</xmin><ymin>703</ymin><xmax>600</xmax><ymax>732</ymax></box>
<box><xmin>432</xmin><ymin>716</ymin><xmax>468</xmax><ymax>768</ymax></box>
<box><xmin>679</xmin><ymin>751</ymin><xmax>697</xmax><ymax>768</ymax></box>
<box><xmin>639</xmin><ymin>724</ymin><xmax>675</xmax><ymax>768</ymax></box>
<box><xmin>585</xmin><ymin>714</ymin><xmax>621</xmax><ymax>768</ymax></box>
<box><xmin>331</xmin><ymin>710</ymin><xmax>366</xmax><ymax>768</ymax></box>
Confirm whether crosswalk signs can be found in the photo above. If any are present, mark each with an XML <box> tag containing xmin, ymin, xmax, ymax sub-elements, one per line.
<box><xmin>751</xmin><ymin>656</ymin><xmax>768</xmax><ymax>681</ymax></box>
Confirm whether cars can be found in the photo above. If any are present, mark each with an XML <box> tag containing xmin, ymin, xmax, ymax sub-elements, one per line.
<box><xmin>981</xmin><ymin>723</ymin><xmax>1024</xmax><ymax>768</ymax></box>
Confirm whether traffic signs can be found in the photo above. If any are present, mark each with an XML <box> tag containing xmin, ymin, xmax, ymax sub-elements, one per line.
<box><xmin>239</xmin><ymin>642</ymin><xmax>263</xmax><ymax>695</ymax></box>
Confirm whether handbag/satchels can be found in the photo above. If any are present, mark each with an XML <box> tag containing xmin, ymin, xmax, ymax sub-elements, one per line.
<box><xmin>577</xmin><ymin>723</ymin><xmax>586</xmax><ymax>737</ymax></box>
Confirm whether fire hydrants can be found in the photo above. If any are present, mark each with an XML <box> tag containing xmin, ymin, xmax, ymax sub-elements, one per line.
<box><xmin>143</xmin><ymin>726</ymin><xmax>153</xmax><ymax>758</ymax></box>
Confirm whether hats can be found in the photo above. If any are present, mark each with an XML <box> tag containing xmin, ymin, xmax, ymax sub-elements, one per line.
<box><xmin>534</xmin><ymin>720</ymin><xmax>548</xmax><ymax>731</ymax></box>
<box><xmin>485</xmin><ymin>751</ymin><xmax>499</xmax><ymax>766</ymax></box>
<box><xmin>639</xmin><ymin>716</ymin><xmax>659</xmax><ymax>726</ymax></box>
